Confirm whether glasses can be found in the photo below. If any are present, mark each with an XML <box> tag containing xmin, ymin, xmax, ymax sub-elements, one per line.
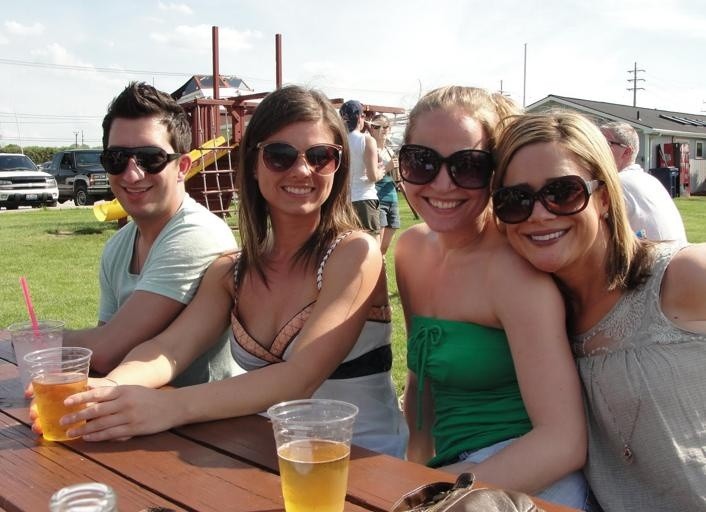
<box><xmin>399</xmin><ymin>144</ymin><xmax>493</xmax><ymax>190</ymax></box>
<box><xmin>256</xmin><ymin>141</ymin><xmax>344</xmax><ymax>176</ymax></box>
<box><xmin>371</xmin><ymin>124</ymin><xmax>389</xmax><ymax>130</ymax></box>
<box><xmin>493</xmin><ymin>176</ymin><xmax>604</xmax><ymax>224</ymax></box>
<box><xmin>100</xmin><ymin>147</ymin><xmax>181</xmax><ymax>174</ymax></box>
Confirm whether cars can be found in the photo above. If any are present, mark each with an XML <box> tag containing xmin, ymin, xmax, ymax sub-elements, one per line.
<box><xmin>35</xmin><ymin>161</ymin><xmax>53</xmax><ymax>170</ymax></box>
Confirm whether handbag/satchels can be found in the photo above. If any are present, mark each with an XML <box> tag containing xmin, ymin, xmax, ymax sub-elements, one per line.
<box><xmin>389</xmin><ymin>473</ymin><xmax>542</xmax><ymax>511</ymax></box>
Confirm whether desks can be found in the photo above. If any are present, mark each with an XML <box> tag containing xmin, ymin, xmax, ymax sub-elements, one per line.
<box><xmin>0</xmin><ymin>330</ymin><xmax>583</xmax><ymax>511</ymax></box>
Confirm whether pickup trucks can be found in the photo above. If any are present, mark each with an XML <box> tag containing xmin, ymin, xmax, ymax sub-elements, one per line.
<box><xmin>43</xmin><ymin>148</ymin><xmax>115</xmax><ymax>207</ymax></box>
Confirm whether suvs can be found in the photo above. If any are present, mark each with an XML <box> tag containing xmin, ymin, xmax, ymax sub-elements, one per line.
<box><xmin>0</xmin><ymin>153</ymin><xmax>61</xmax><ymax>212</ymax></box>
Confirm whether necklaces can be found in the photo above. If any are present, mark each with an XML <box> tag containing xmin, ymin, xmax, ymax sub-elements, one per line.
<box><xmin>578</xmin><ymin>292</ymin><xmax>651</xmax><ymax>463</ymax></box>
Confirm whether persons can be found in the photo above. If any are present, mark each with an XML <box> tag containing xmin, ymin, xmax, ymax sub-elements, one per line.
<box><xmin>338</xmin><ymin>100</ymin><xmax>387</xmax><ymax>252</ymax></box>
<box><xmin>22</xmin><ymin>83</ymin><xmax>410</xmax><ymax>462</ymax></box>
<box><xmin>11</xmin><ymin>82</ymin><xmax>248</xmax><ymax>387</ymax></box>
<box><xmin>490</xmin><ymin>108</ymin><xmax>705</xmax><ymax>511</ymax></box>
<box><xmin>392</xmin><ymin>86</ymin><xmax>593</xmax><ymax>511</ymax></box>
<box><xmin>370</xmin><ymin>115</ymin><xmax>404</xmax><ymax>256</ymax></box>
<box><xmin>598</xmin><ymin>122</ymin><xmax>686</xmax><ymax>246</ymax></box>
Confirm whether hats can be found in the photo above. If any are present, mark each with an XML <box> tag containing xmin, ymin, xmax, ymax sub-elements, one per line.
<box><xmin>339</xmin><ymin>100</ymin><xmax>361</xmax><ymax>132</ymax></box>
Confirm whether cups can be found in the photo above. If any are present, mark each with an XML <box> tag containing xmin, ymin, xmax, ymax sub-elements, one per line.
<box><xmin>6</xmin><ymin>319</ymin><xmax>94</xmax><ymax>442</ymax></box>
<box><xmin>48</xmin><ymin>482</ymin><xmax>119</xmax><ymax>512</ymax></box>
<box><xmin>267</xmin><ymin>399</ymin><xmax>358</xmax><ymax>512</ymax></box>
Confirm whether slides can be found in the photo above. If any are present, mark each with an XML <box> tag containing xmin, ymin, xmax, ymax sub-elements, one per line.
<box><xmin>93</xmin><ymin>136</ymin><xmax>237</xmax><ymax>222</ymax></box>
